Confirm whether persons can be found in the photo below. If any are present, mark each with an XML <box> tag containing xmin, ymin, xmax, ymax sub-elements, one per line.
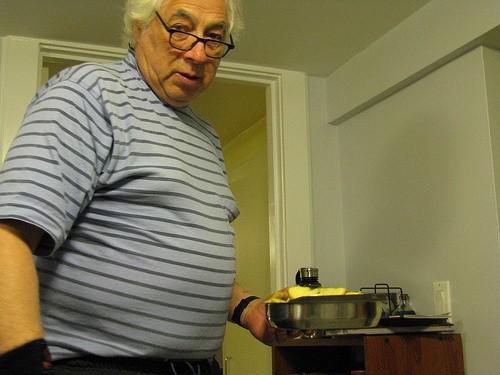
<box><xmin>0</xmin><ymin>0</ymin><xmax>316</xmax><ymax>375</ymax></box>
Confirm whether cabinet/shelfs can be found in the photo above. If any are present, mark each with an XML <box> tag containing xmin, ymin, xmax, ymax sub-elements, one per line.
<box><xmin>272</xmin><ymin>333</ymin><xmax>465</xmax><ymax>375</ymax></box>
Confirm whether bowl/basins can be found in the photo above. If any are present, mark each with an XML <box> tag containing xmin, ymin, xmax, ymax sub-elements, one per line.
<box><xmin>258</xmin><ymin>292</ymin><xmax>387</xmax><ymax>329</ymax></box>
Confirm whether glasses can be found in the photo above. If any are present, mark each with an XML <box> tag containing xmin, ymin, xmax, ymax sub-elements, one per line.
<box><xmin>155</xmin><ymin>11</ymin><xmax>235</xmax><ymax>59</ymax></box>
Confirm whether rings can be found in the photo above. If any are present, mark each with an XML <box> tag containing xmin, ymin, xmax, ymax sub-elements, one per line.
<box><xmin>289</xmin><ymin>332</ymin><xmax>303</xmax><ymax>340</ymax></box>
<box><xmin>302</xmin><ymin>331</ymin><xmax>316</xmax><ymax>340</ymax></box>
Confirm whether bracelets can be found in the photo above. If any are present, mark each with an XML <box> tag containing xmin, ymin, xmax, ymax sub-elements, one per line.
<box><xmin>0</xmin><ymin>338</ymin><xmax>50</xmax><ymax>375</ymax></box>
<box><xmin>232</xmin><ymin>295</ymin><xmax>261</xmax><ymax>328</ymax></box>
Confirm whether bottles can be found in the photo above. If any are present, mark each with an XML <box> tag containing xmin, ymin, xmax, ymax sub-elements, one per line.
<box><xmin>297</xmin><ymin>267</ymin><xmax>326</xmax><ymax>339</ymax></box>
<box><xmin>391</xmin><ymin>293</ymin><xmax>417</xmax><ymax>316</ymax></box>
